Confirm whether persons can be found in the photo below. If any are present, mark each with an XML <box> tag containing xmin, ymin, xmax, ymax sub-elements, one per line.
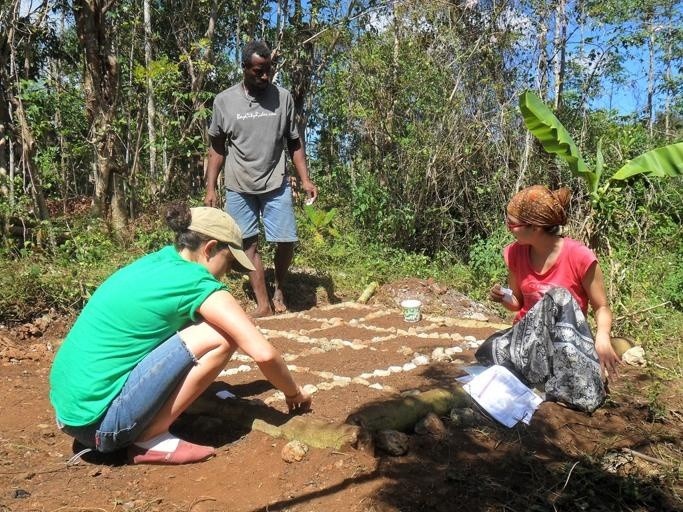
<box><xmin>203</xmin><ymin>43</ymin><xmax>320</xmax><ymax>317</ymax></box>
<box><xmin>475</xmin><ymin>185</ymin><xmax>647</xmax><ymax>413</ymax></box>
<box><xmin>49</xmin><ymin>204</ymin><xmax>313</xmax><ymax>465</ymax></box>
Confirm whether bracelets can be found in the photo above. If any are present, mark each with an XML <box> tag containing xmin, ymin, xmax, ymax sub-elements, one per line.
<box><xmin>286</xmin><ymin>391</ymin><xmax>301</xmax><ymax>398</ymax></box>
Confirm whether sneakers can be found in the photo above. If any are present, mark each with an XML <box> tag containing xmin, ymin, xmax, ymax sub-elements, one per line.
<box><xmin>128</xmin><ymin>440</ymin><xmax>215</xmax><ymax>464</ymax></box>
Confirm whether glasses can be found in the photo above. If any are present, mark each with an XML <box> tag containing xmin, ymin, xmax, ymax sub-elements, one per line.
<box><xmin>507</xmin><ymin>222</ymin><xmax>530</xmax><ymax>232</ymax></box>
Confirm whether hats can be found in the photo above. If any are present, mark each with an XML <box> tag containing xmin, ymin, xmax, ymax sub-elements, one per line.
<box><xmin>186</xmin><ymin>207</ymin><xmax>256</xmax><ymax>272</ymax></box>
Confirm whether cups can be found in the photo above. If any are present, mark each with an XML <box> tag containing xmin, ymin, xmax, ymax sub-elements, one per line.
<box><xmin>402</xmin><ymin>299</ymin><xmax>423</xmax><ymax>323</ymax></box>
